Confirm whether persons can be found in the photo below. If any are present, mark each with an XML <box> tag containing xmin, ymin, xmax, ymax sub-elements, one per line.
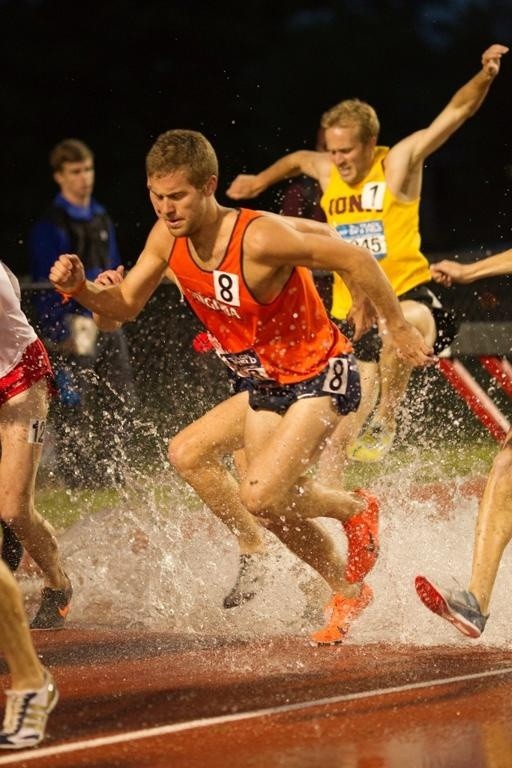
<box><xmin>50</xmin><ymin>43</ymin><xmax>512</xmax><ymax>648</ymax></box>
<box><xmin>1</xmin><ymin>261</ymin><xmax>72</xmax><ymax>750</ymax></box>
<box><xmin>25</xmin><ymin>137</ymin><xmax>132</xmax><ymax>489</ymax></box>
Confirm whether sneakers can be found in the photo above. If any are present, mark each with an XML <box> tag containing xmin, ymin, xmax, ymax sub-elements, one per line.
<box><xmin>343</xmin><ymin>486</ymin><xmax>379</xmax><ymax>583</ymax></box>
<box><xmin>346</xmin><ymin>422</ymin><xmax>396</xmax><ymax>463</ymax></box>
<box><xmin>0</xmin><ymin>664</ymin><xmax>58</xmax><ymax>748</ymax></box>
<box><xmin>223</xmin><ymin>552</ymin><xmax>283</xmax><ymax>608</ymax></box>
<box><xmin>311</xmin><ymin>579</ymin><xmax>374</xmax><ymax>642</ymax></box>
<box><xmin>415</xmin><ymin>575</ymin><xmax>489</xmax><ymax>637</ymax></box>
<box><xmin>29</xmin><ymin>572</ymin><xmax>72</xmax><ymax>631</ymax></box>
